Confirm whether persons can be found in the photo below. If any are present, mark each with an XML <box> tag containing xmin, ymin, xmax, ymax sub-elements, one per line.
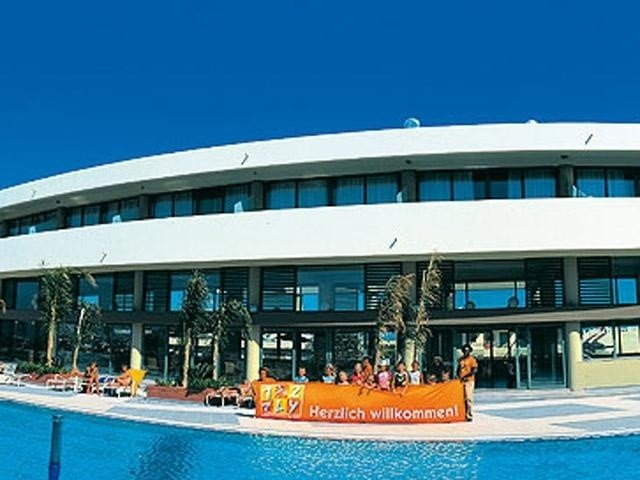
<box><xmin>390</xmin><ymin>361</ymin><xmax>411</xmax><ymax>396</ymax></box>
<box><xmin>455</xmin><ymin>343</ymin><xmax>479</xmax><ymax>421</ymax></box>
<box><xmin>82</xmin><ymin>360</ymin><xmax>101</xmax><ymax>396</ymax></box>
<box><xmin>207</xmin><ymin>379</ymin><xmax>254</xmax><ymax>404</ymax></box>
<box><xmin>425</xmin><ymin>354</ymin><xmax>450</xmax><ymax>386</ymax></box>
<box><xmin>350</xmin><ymin>360</ymin><xmax>365</xmax><ymax>386</ymax></box>
<box><xmin>362</xmin><ymin>356</ymin><xmax>373</xmax><ymax>381</ymax></box>
<box><xmin>293</xmin><ymin>366</ymin><xmax>309</xmax><ymax>383</ymax></box>
<box><xmin>376</xmin><ymin>359</ymin><xmax>393</xmax><ymax>391</ymax></box>
<box><xmin>107</xmin><ymin>364</ymin><xmax>133</xmax><ymax>388</ymax></box>
<box><xmin>253</xmin><ymin>367</ymin><xmax>275</xmax><ymax>383</ymax></box>
<box><xmin>506</xmin><ymin>355</ymin><xmax>515</xmax><ymax>389</ymax></box>
<box><xmin>320</xmin><ymin>362</ymin><xmax>336</xmax><ymax>384</ymax></box>
<box><xmin>337</xmin><ymin>371</ymin><xmax>349</xmax><ymax>385</ymax></box>
<box><xmin>364</xmin><ymin>374</ymin><xmax>378</xmax><ymax>390</ymax></box>
<box><xmin>410</xmin><ymin>359</ymin><xmax>423</xmax><ymax>386</ymax></box>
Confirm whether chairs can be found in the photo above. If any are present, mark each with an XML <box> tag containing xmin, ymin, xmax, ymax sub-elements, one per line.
<box><xmin>2</xmin><ymin>371</ymin><xmax>252</xmax><ymax>409</ymax></box>
